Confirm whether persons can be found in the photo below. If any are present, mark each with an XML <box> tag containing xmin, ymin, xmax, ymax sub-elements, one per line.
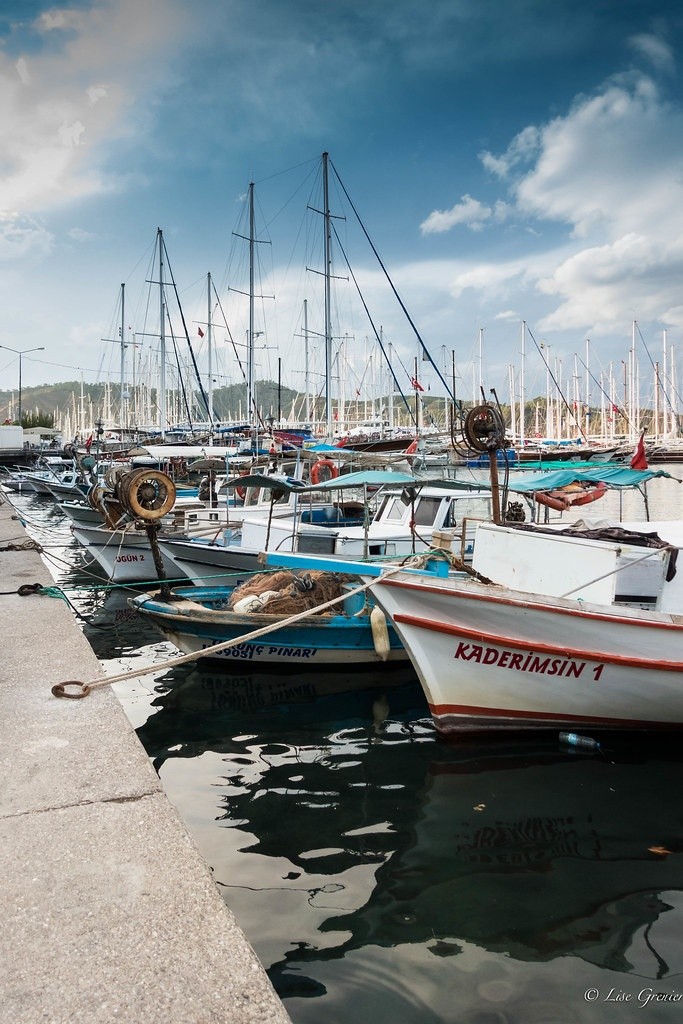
<box><xmin>198</xmin><ymin>470</ymin><xmax>219</xmax><ymax>519</ymax></box>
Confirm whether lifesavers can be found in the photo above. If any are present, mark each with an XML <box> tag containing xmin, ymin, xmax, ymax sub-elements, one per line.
<box><xmin>535</xmin><ymin>433</ymin><xmax>540</xmax><ymax>438</ymax></box>
<box><xmin>372</xmin><ymin>432</ymin><xmax>378</xmax><ymax>439</ymax></box>
<box><xmin>236</xmin><ymin>472</ymin><xmax>261</xmax><ymax>501</ymax></box>
<box><xmin>352</xmin><ymin>434</ymin><xmax>368</xmax><ymax>443</ymax></box>
<box><xmin>535</xmin><ymin>494</ymin><xmax>567</xmax><ymax>511</ymax></box>
<box><xmin>177</xmin><ymin>459</ymin><xmax>188</xmax><ymax>476</ymax></box>
<box><xmin>571</xmin><ymin>482</ymin><xmax>608</xmax><ymax>506</ymax></box>
<box><xmin>310</xmin><ymin>460</ymin><xmax>338</xmax><ymax>484</ymax></box>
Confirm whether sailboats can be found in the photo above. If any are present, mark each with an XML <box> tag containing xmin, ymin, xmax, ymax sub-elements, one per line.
<box><xmin>0</xmin><ymin>150</ymin><xmax>682</xmax><ymax>735</ymax></box>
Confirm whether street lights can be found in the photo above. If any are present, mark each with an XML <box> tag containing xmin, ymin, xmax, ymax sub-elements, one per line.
<box><xmin>0</xmin><ymin>344</ymin><xmax>45</xmax><ymax>428</ymax></box>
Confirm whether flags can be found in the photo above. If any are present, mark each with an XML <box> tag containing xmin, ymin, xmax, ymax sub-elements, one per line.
<box><xmin>412</xmin><ymin>378</ymin><xmax>424</xmax><ymax>391</ymax></box>
<box><xmin>85</xmin><ymin>435</ymin><xmax>92</xmax><ymax>447</ymax></box>
<box><xmin>629</xmin><ymin>436</ymin><xmax>648</xmax><ymax>469</ymax></box>
<box><xmin>198</xmin><ymin>328</ymin><xmax>204</xmax><ymax>337</ymax></box>
